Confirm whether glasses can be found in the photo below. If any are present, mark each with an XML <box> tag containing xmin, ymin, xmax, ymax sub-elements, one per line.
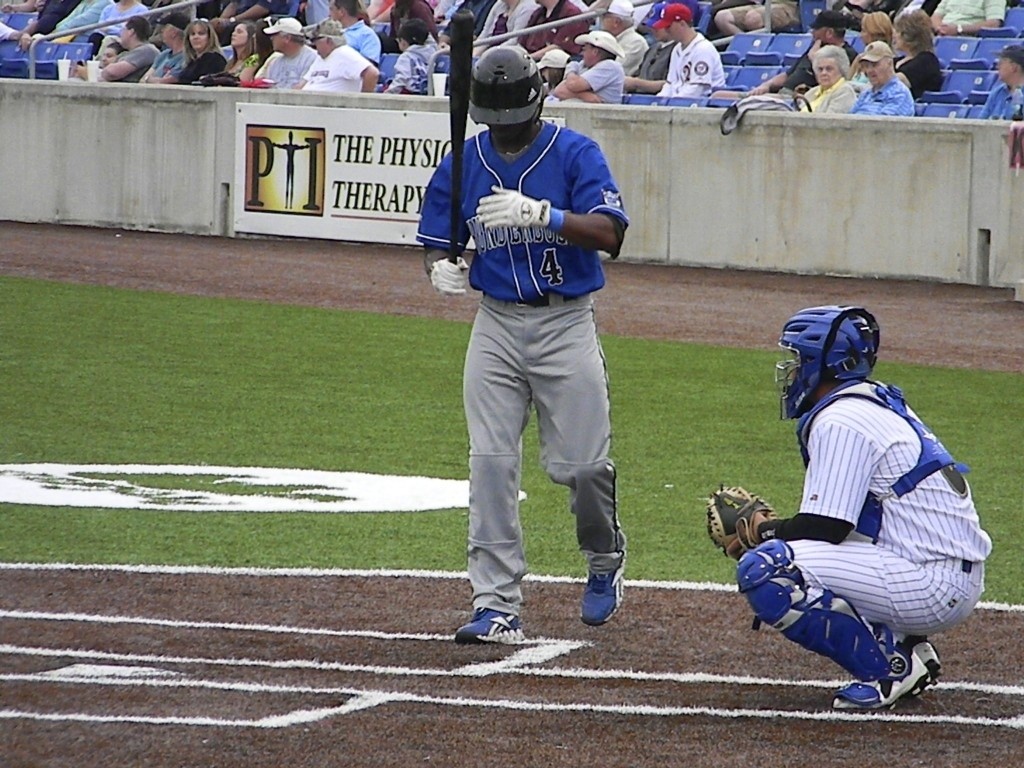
<box><xmin>310</xmin><ymin>37</ymin><xmax>324</xmax><ymax>42</ymax></box>
<box><xmin>192</xmin><ymin>18</ymin><xmax>209</xmax><ymax>23</ymax></box>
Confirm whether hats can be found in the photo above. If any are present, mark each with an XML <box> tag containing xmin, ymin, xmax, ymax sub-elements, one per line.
<box><xmin>575</xmin><ymin>31</ymin><xmax>626</xmax><ymax>59</ymax></box>
<box><xmin>608</xmin><ymin>0</ymin><xmax>635</xmax><ymax>17</ymax></box>
<box><xmin>652</xmin><ymin>4</ymin><xmax>692</xmax><ymax>29</ymax></box>
<box><xmin>263</xmin><ymin>18</ymin><xmax>305</xmax><ymax>36</ymax></box>
<box><xmin>536</xmin><ymin>49</ymin><xmax>571</xmax><ymax>69</ymax></box>
<box><xmin>990</xmin><ymin>44</ymin><xmax>1024</xmax><ymax>64</ymax></box>
<box><xmin>156</xmin><ymin>13</ymin><xmax>190</xmax><ymax>30</ymax></box>
<box><xmin>399</xmin><ymin>17</ymin><xmax>429</xmax><ymax>46</ymax></box>
<box><xmin>858</xmin><ymin>41</ymin><xmax>893</xmax><ymax>62</ymax></box>
<box><xmin>306</xmin><ymin>19</ymin><xmax>344</xmax><ymax>38</ymax></box>
<box><xmin>809</xmin><ymin>10</ymin><xmax>846</xmax><ymax>29</ymax></box>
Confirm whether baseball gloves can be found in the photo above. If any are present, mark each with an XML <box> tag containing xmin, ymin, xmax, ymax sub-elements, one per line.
<box><xmin>704</xmin><ymin>483</ymin><xmax>775</xmax><ymax>559</ymax></box>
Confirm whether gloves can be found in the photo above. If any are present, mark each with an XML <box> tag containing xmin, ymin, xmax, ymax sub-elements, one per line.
<box><xmin>476</xmin><ymin>185</ymin><xmax>550</xmax><ymax>228</ymax></box>
<box><xmin>431</xmin><ymin>257</ymin><xmax>469</xmax><ymax>295</ymax></box>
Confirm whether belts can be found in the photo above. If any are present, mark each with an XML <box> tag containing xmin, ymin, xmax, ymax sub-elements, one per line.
<box><xmin>512</xmin><ymin>292</ymin><xmax>578</xmax><ymax>307</ymax></box>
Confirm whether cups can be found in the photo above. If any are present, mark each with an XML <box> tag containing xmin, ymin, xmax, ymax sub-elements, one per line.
<box><xmin>87</xmin><ymin>61</ymin><xmax>100</xmax><ymax>82</ymax></box>
<box><xmin>432</xmin><ymin>74</ymin><xmax>448</xmax><ymax>96</ymax></box>
<box><xmin>57</xmin><ymin>59</ymin><xmax>70</xmax><ymax>82</ymax></box>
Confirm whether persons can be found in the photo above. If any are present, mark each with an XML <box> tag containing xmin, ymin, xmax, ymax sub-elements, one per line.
<box><xmin>411</xmin><ymin>49</ymin><xmax>635</xmax><ymax>642</ymax></box>
<box><xmin>0</xmin><ymin>0</ymin><xmax>1002</xmax><ymax>116</ymax></box>
<box><xmin>730</xmin><ymin>300</ymin><xmax>993</xmax><ymax>711</ymax></box>
<box><xmin>978</xmin><ymin>44</ymin><xmax>1024</xmax><ymax>121</ymax></box>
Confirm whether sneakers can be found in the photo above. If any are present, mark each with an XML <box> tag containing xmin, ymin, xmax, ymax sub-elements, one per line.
<box><xmin>456</xmin><ymin>607</ymin><xmax>525</xmax><ymax>643</ymax></box>
<box><xmin>904</xmin><ymin>636</ymin><xmax>942</xmax><ymax>697</ymax></box>
<box><xmin>581</xmin><ymin>558</ymin><xmax>623</xmax><ymax>625</ymax></box>
<box><xmin>834</xmin><ymin>646</ymin><xmax>932</xmax><ymax>710</ymax></box>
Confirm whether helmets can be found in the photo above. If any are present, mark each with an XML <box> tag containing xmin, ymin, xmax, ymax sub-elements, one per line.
<box><xmin>470</xmin><ymin>46</ymin><xmax>542</xmax><ymax>124</ymax></box>
<box><xmin>775</xmin><ymin>306</ymin><xmax>879</xmax><ymax>420</ymax></box>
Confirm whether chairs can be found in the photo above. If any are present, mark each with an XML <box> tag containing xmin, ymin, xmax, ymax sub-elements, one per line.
<box><xmin>0</xmin><ymin>1</ymin><xmax>480</xmax><ymax>98</ymax></box>
<box><xmin>622</xmin><ymin>0</ymin><xmax>1024</xmax><ymax>120</ymax></box>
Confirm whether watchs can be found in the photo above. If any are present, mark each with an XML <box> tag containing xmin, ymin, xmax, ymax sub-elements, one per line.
<box><xmin>145</xmin><ymin>76</ymin><xmax>149</xmax><ymax>83</ymax></box>
<box><xmin>956</xmin><ymin>24</ymin><xmax>962</xmax><ymax>34</ymax></box>
<box><xmin>229</xmin><ymin>17</ymin><xmax>238</xmax><ymax>23</ymax></box>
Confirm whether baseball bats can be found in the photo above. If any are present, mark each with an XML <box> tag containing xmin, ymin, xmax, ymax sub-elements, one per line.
<box><xmin>449</xmin><ymin>8</ymin><xmax>476</xmax><ymax>265</ymax></box>
